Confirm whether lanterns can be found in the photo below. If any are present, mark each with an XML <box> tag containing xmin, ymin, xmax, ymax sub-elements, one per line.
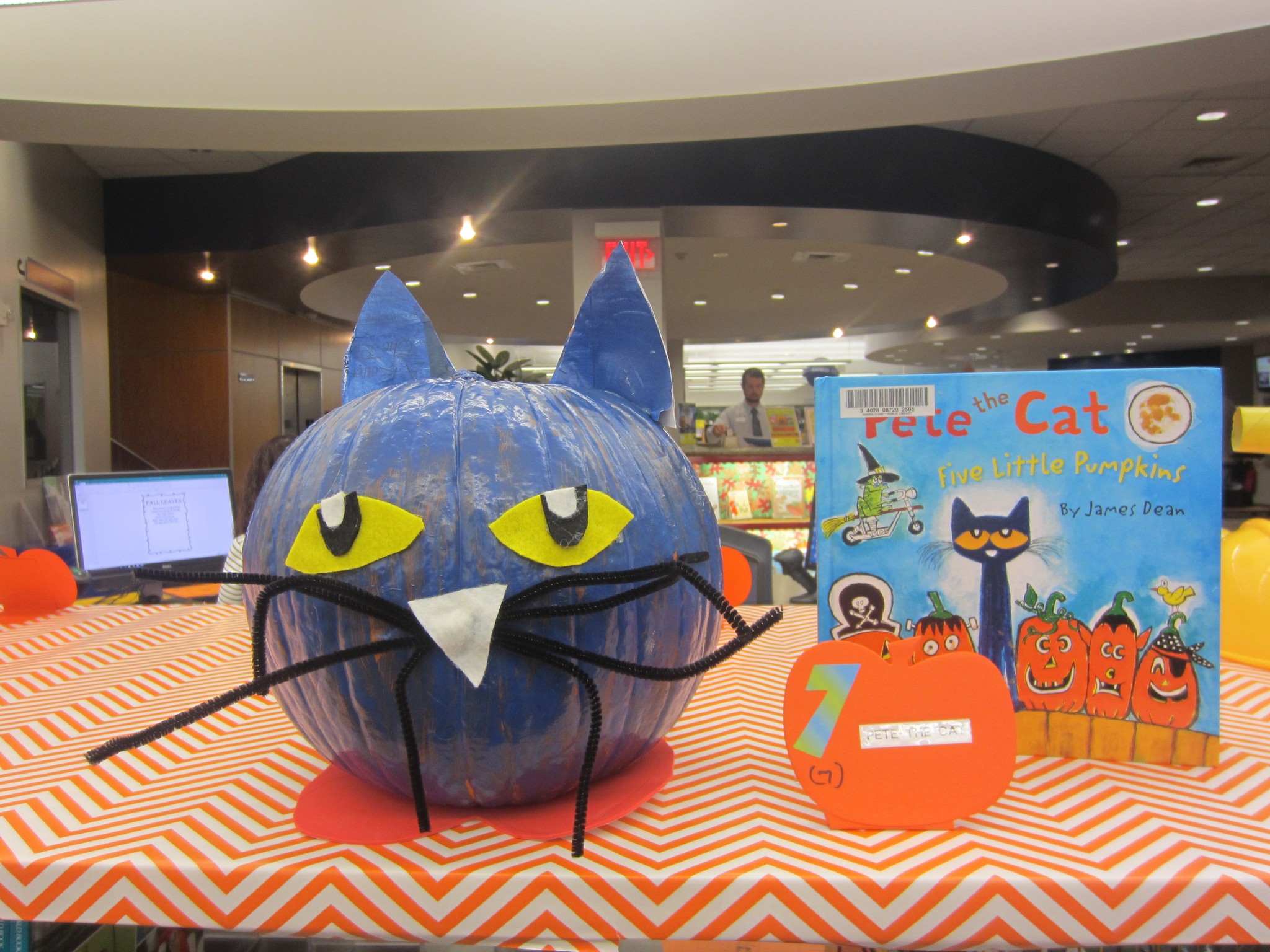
<box><xmin>230</xmin><ymin>242</ymin><xmax>727</xmax><ymax>813</ymax></box>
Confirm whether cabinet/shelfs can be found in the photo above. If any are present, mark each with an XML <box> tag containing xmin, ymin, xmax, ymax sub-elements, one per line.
<box><xmin>678</xmin><ymin>445</ymin><xmax>818</xmax><ymax>571</ymax></box>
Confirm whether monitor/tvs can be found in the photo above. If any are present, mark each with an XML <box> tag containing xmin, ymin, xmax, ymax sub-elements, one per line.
<box><xmin>1254</xmin><ymin>352</ymin><xmax>1270</xmax><ymax>393</ymax></box>
<box><xmin>804</xmin><ymin>482</ymin><xmax>818</xmax><ymax>567</ymax></box>
<box><xmin>66</xmin><ymin>468</ymin><xmax>240</xmax><ymax>579</ymax></box>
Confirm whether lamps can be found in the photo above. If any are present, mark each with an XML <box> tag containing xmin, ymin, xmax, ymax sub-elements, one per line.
<box><xmin>198</xmin><ymin>213</ymin><xmax>476</xmax><ymax>283</ymax></box>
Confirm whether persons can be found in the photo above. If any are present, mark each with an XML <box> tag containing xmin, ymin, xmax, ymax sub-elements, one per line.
<box><xmin>705</xmin><ymin>366</ymin><xmax>774</xmax><ymax>447</ymax></box>
<box><xmin>210</xmin><ymin>434</ymin><xmax>293</xmax><ymax>605</ymax></box>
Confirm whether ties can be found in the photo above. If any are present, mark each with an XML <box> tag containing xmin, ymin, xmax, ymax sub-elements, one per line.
<box><xmin>751</xmin><ymin>408</ymin><xmax>763</xmax><ymax>436</ymax></box>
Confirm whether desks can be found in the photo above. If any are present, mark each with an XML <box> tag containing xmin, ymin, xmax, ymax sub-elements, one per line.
<box><xmin>0</xmin><ymin>603</ymin><xmax>1269</xmax><ymax>952</ymax></box>
